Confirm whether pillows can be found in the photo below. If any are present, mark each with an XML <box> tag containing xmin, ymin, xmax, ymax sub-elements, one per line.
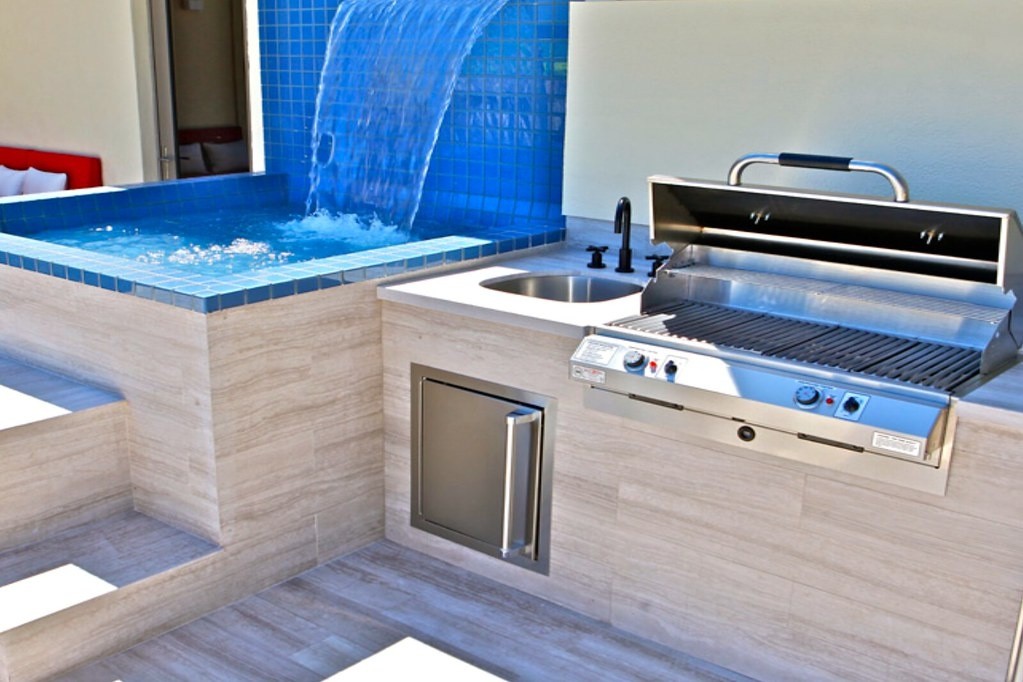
<box><xmin>203</xmin><ymin>139</ymin><xmax>248</xmax><ymax>173</ymax></box>
<box><xmin>179</xmin><ymin>142</ymin><xmax>209</xmax><ymax>175</ymax></box>
<box><xmin>0</xmin><ymin>164</ymin><xmax>26</xmax><ymax>196</ymax></box>
<box><xmin>22</xmin><ymin>167</ymin><xmax>66</xmax><ymax>194</ymax></box>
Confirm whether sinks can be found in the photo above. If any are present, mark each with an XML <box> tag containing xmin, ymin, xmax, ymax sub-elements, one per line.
<box><xmin>476</xmin><ymin>267</ymin><xmax>646</xmax><ymax>304</ymax></box>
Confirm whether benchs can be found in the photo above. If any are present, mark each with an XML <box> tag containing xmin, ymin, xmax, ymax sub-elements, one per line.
<box><xmin>178</xmin><ymin>127</ymin><xmax>242</xmax><ymax>173</ymax></box>
<box><xmin>0</xmin><ymin>146</ymin><xmax>103</xmax><ymax>190</ymax></box>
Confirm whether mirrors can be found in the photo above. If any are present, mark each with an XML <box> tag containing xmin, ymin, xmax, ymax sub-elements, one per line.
<box><xmin>165</xmin><ymin>0</ymin><xmax>252</xmax><ymax>180</ymax></box>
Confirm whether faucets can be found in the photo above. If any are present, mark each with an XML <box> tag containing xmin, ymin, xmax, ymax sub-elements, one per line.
<box><xmin>614</xmin><ymin>196</ymin><xmax>635</xmax><ymax>273</ymax></box>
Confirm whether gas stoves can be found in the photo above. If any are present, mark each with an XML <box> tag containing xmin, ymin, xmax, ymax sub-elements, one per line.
<box><xmin>568</xmin><ymin>152</ymin><xmax>1023</xmax><ymax>476</ymax></box>
